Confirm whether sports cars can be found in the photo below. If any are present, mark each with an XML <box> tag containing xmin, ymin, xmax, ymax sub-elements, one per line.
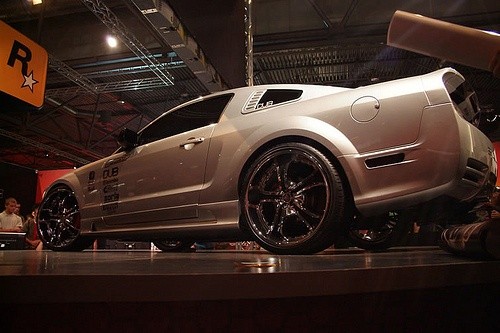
<box><xmin>35</xmin><ymin>65</ymin><xmax>498</xmax><ymax>257</ymax></box>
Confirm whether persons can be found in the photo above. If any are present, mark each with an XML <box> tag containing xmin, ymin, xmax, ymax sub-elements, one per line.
<box><xmin>0</xmin><ymin>197</ymin><xmax>44</xmax><ymax>251</ymax></box>
